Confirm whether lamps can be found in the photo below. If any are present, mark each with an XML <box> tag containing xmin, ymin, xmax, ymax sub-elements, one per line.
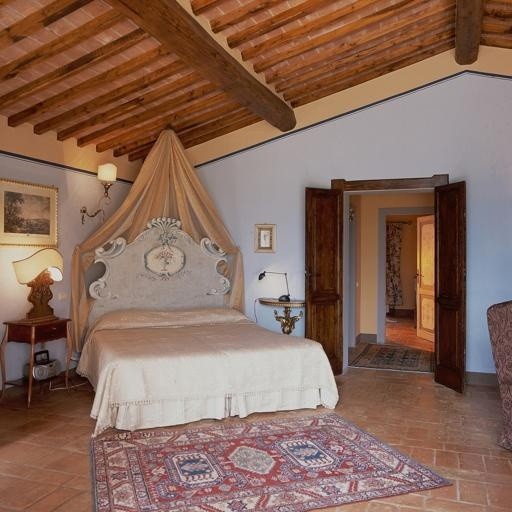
<box><xmin>12</xmin><ymin>248</ymin><xmax>65</xmax><ymax>323</ymax></box>
<box><xmin>259</xmin><ymin>270</ymin><xmax>291</xmax><ymax>303</ymax></box>
<box><xmin>80</xmin><ymin>163</ymin><xmax>118</xmax><ymax>224</ymax></box>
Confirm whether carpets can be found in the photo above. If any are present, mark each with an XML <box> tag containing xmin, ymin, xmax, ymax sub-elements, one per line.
<box><xmin>88</xmin><ymin>410</ymin><xmax>454</xmax><ymax>511</ymax></box>
<box><xmin>348</xmin><ymin>342</ymin><xmax>435</xmax><ymax>375</ymax></box>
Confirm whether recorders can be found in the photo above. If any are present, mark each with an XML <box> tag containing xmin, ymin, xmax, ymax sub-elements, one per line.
<box><xmin>24</xmin><ymin>350</ymin><xmax>61</xmax><ymax>380</ymax></box>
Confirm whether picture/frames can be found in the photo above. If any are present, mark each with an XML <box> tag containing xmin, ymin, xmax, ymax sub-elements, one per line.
<box><xmin>1</xmin><ymin>178</ymin><xmax>58</xmax><ymax>249</ymax></box>
<box><xmin>254</xmin><ymin>223</ymin><xmax>277</xmax><ymax>252</ymax></box>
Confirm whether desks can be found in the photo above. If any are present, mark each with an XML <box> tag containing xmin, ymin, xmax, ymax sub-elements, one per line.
<box><xmin>259</xmin><ymin>298</ymin><xmax>306</xmax><ymax>335</ymax></box>
<box><xmin>0</xmin><ymin>318</ymin><xmax>73</xmax><ymax>409</ymax></box>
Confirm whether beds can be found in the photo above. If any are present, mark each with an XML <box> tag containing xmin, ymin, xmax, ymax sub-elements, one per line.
<box><xmin>79</xmin><ymin>217</ymin><xmax>322</xmax><ymax>431</ymax></box>
